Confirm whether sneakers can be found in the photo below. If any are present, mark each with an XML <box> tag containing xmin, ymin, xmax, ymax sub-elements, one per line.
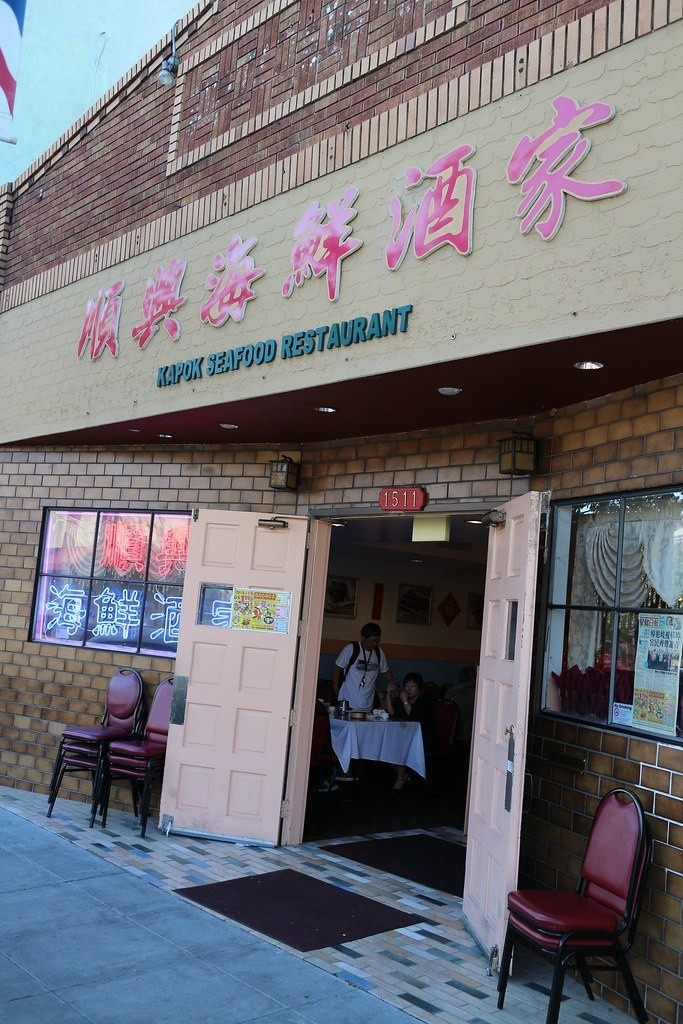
<box><xmin>333</xmin><ymin>770</ymin><xmax>358</xmax><ymax>782</ymax></box>
<box><xmin>313</xmin><ymin>780</ymin><xmax>338</xmax><ymax>792</ymax></box>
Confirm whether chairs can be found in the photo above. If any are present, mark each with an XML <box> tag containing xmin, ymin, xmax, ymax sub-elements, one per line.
<box><xmin>422</xmin><ymin>682</ymin><xmax>459</xmax><ymax>789</ymax></box>
<box><xmin>311</xmin><ymin>679</ymin><xmax>359</xmax><ymax>795</ymax></box>
<box><xmin>497</xmin><ymin>788</ymin><xmax>650</xmax><ymax>1023</ymax></box>
<box><xmin>47</xmin><ymin>668</ymin><xmax>174</xmax><ymax>839</ymax></box>
<box><xmin>497</xmin><ymin>818</ymin><xmax>653</xmax><ymax>1024</ymax></box>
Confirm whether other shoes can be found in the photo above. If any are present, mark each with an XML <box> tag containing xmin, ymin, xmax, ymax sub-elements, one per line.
<box><xmin>390</xmin><ymin>777</ymin><xmax>406</xmax><ymax>792</ymax></box>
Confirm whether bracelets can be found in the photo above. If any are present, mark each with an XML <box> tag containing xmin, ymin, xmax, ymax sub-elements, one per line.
<box><xmin>404</xmin><ymin>702</ymin><xmax>408</xmax><ymax>705</ymax></box>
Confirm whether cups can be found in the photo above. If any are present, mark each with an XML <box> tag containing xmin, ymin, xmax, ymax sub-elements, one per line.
<box><xmin>339</xmin><ymin>698</ymin><xmax>349</xmax><ymax>715</ymax></box>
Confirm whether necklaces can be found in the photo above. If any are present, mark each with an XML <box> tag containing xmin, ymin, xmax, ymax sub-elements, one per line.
<box><xmin>358</xmin><ymin>643</ymin><xmax>374</xmax><ymax>689</ymax></box>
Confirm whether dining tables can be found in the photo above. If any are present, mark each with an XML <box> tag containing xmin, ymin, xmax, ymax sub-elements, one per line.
<box><xmin>326</xmin><ymin>716</ymin><xmax>427</xmax><ymax>791</ymax></box>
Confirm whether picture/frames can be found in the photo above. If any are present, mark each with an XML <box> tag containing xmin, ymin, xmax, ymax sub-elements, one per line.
<box><xmin>466</xmin><ymin>591</ymin><xmax>484</xmax><ymax>629</ymax></box>
<box><xmin>396</xmin><ymin>583</ymin><xmax>432</xmax><ymax>625</ymax></box>
<box><xmin>324</xmin><ymin>574</ymin><xmax>358</xmax><ymax>618</ymax></box>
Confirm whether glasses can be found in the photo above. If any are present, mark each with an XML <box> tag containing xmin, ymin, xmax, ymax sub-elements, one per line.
<box><xmin>367</xmin><ymin>639</ymin><xmax>381</xmax><ymax>643</ymax></box>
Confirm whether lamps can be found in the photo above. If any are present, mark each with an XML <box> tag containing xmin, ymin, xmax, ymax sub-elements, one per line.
<box><xmin>496</xmin><ymin>431</ymin><xmax>537</xmax><ymax>476</ymax></box>
<box><xmin>267</xmin><ymin>454</ymin><xmax>299</xmax><ymax>490</ymax></box>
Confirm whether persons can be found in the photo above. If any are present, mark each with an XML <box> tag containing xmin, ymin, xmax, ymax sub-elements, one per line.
<box><xmin>334</xmin><ymin>622</ymin><xmax>393</xmax><ymax>717</ymax></box>
<box><xmin>313</xmin><ymin>762</ymin><xmax>361</xmax><ymax>792</ymax></box>
<box><xmin>384</xmin><ymin>672</ymin><xmax>443</xmax><ymax>794</ymax></box>
